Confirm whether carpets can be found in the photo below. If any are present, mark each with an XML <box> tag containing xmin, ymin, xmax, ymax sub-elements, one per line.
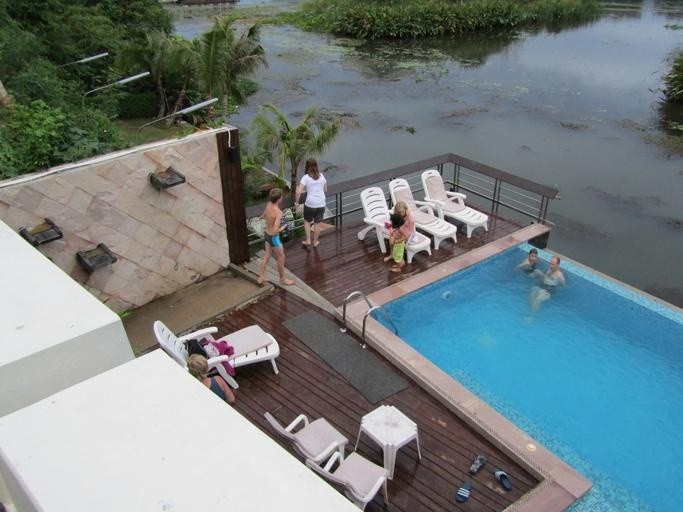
<box><xmin>281</xmin><ymin>308</ymin><xmax>411</xmax><ymax>405</ymax></box>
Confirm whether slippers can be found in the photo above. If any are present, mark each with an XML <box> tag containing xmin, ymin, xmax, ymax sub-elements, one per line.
<box><xmin>470</xmin><ymin>455</ymin><xmax>486</xmax><ymax>474</ymax></box>
<box><xmin>456</xmin><ymin>480</ymin><xmax>472</xmax><ymax>502</ymax></box>
<box><xmin>492</xmin><ymin>468</ymin><xmax>511</xmax><ymax>490</ymax></box>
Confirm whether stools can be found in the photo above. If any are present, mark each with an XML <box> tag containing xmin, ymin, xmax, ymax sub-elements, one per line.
<box><xmin>354</xmin><ymin>404</ymin><xmax>422</xmax><ymax>480</ymax></box>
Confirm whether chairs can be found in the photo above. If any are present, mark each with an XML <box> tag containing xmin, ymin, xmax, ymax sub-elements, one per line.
<box><xmin>150</xmin><ymin>317</ymin><xmax>281</xmax><ymax>390</ymax></box>
<box><xmin>264</xmin><ymin>408</ymin><xmax>390</xmax><ymax>512</ymax></box>
<box><xmin>355</xmin><ymin>167</ymin><xmax>488</xmax><ymax>264</ymax></box>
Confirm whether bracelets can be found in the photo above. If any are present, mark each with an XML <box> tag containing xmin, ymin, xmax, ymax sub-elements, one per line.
<box><xmin>294</xmin><ymin>202</ymin><xmax>298</xmax><ymax>206</ymax></box>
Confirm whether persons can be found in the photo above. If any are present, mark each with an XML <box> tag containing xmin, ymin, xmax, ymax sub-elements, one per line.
<box><xmin>255</xmin><ymin>186</ymin><xmax>296</xmax><ymax>286</ymax></box>
<box><xmin>385</xmin><ymin>213</ymin><xmax>408</xmax><ymax>272</ymax></box>
<box><xmin>516</xmin><ymin>249</ymin><xmax>543</xmax><ymax>280</ymax></box>
<box><xmin>292</xmin><ymin>157</ymin><xmax>327</xmax><ymax>247</ymax></box>
<box><xmin>530</xmin><ymin>257</ymin><xmax>566</xmax><ymax>310</ymax></box>
<box><xmin>183</xmin><ymin>353</ymin><xmax>237</xmax><ymax>408</ymax></box>
<box><xmin>381</xmin><ymin>201</ymin><xmax>415</xmax><ymax>268</ymax></box>
